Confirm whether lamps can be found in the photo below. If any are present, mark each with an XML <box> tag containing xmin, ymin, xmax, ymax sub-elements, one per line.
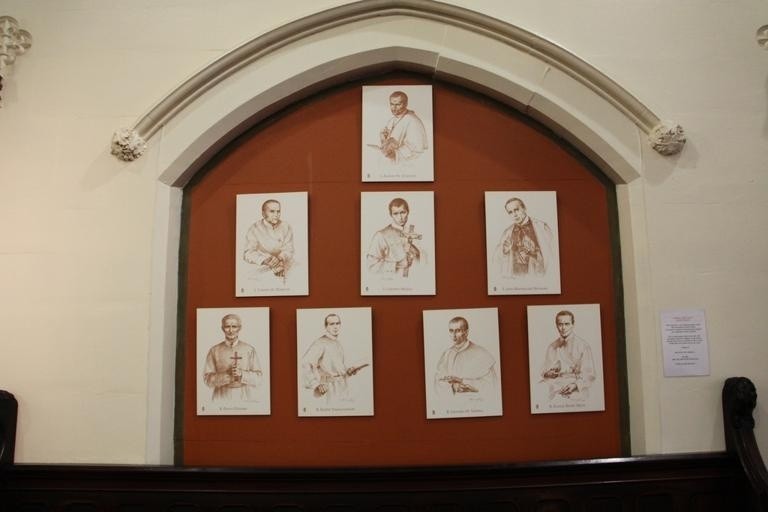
<box><xmin>0</xmin><ymin>12</ymin><xmax>33</xmax><ymax>107</ymax></box>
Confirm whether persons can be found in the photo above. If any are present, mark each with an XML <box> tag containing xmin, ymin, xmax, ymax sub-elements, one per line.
<box><xmin>366</xmin><ymin>91</ymin><xmax>429</xmax><ymax>166</ymax></box>
<box><xmin>298</xmin><ymin>314</ymin><xmax>351</xmax><ymax>402</ymax></box>
<box><xmin>494</xmin><ymin>198</ymin><xmax>554</xmax><ymax>281</ymax></box>
<box><xmin>201</xmin><ymin>315</ymin><xmax>263</xmax><ymax>406</ymax></box>
<box><xmin>434</xmin><ymin>315</ymin><xmax>499</xmax><ymax>407</ymax></box>
<box><xmin>364</xmin><ymin>197</ymin><xmax>423</xmax><ymax>279</ymax></box>
<box><xmin>537</xmin><ymin>311</ymin><xmax>596</xmax><ymax>403</ymax></box>
<box><xmin>242</xmin><ymin>200</ymin><xmax>294</xmax><ymax>285</ymax></box>
